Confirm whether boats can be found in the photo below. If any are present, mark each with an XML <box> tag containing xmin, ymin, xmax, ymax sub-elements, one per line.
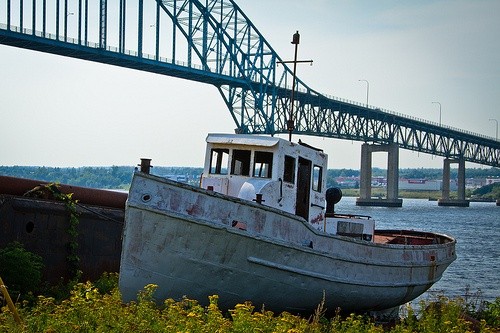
<box><xmin>116</xmin><ymin>30</ymin><xmax>457</xmax><ymax>322</ymax></box>
<box><xmin>0</xmin><ymin>176</ymin><xmax>130</xmax><ymax>306</ymax></box>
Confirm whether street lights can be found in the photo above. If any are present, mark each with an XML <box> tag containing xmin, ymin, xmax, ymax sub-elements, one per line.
<box><xmin>489</xmin><ymin>118</ymin><xmax>498</xmax><ymax>140</ymax></box>
<box><xmin>431</xmin><ymin>101</ymin><xmax>442</xmax><ymax>127</ymax></box>
<box><xmin>357</xmin><ymin>79</ymin><xmax>370</xmax><ymax>108</ymax></box>
